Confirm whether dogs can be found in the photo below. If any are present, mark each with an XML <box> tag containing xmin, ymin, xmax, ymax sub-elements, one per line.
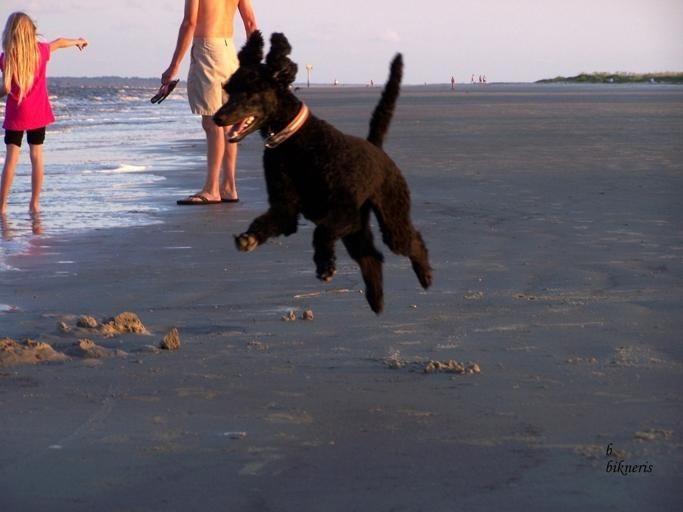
<box><xmin>211</xmin><ymin>28</ymin><xmax>434</xmax><ymax>316</ymax></box>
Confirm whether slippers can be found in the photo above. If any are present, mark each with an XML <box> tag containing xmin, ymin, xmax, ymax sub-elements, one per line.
<box><xmin>175</xmin><ymin>193</ymin><xmax>221</xmax><ymax>206</ymax></box>
<box><xmin>149</xmin><ymin>78</ymin><xmax>180</xmax><ymax>105</ymax></box>
<box><xmin>220</xmin><ymin>196</ymin><xmax>239</xmax><ymax>203</ymax></box>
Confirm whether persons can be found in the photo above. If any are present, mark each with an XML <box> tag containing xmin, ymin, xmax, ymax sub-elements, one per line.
<box><xmin>451</xmin><ymin>76</ymin><xmax>456</xmax><ymax>88</ymax></box>
<box><xmin>0</xmin><ymin>12</ymin><xmax>88</xmax><ymax>214</ymax></box>
<box><xmin>0</xmin><ymin>213</ymin><xmax>43</xmax><ymax>239</ymax></box>
<box><xmin>472</xmin><ymin>73</ymin><xmax>487</xmax><ymax>83</ymax></box>
<box><xmin>161</xmin><ymin>0</ymin><xmax>258</xmax><ymax>205</ymax></box>
